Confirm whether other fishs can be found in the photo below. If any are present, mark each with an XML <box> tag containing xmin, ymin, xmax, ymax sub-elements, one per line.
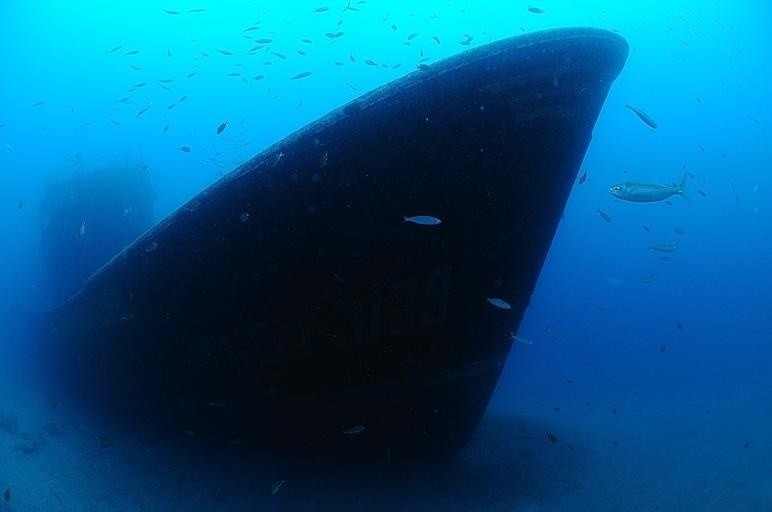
<box><xmin>110</xmin><ymin>0</ymin><xmax>474</xmax><ymax>153</ymax></box>
